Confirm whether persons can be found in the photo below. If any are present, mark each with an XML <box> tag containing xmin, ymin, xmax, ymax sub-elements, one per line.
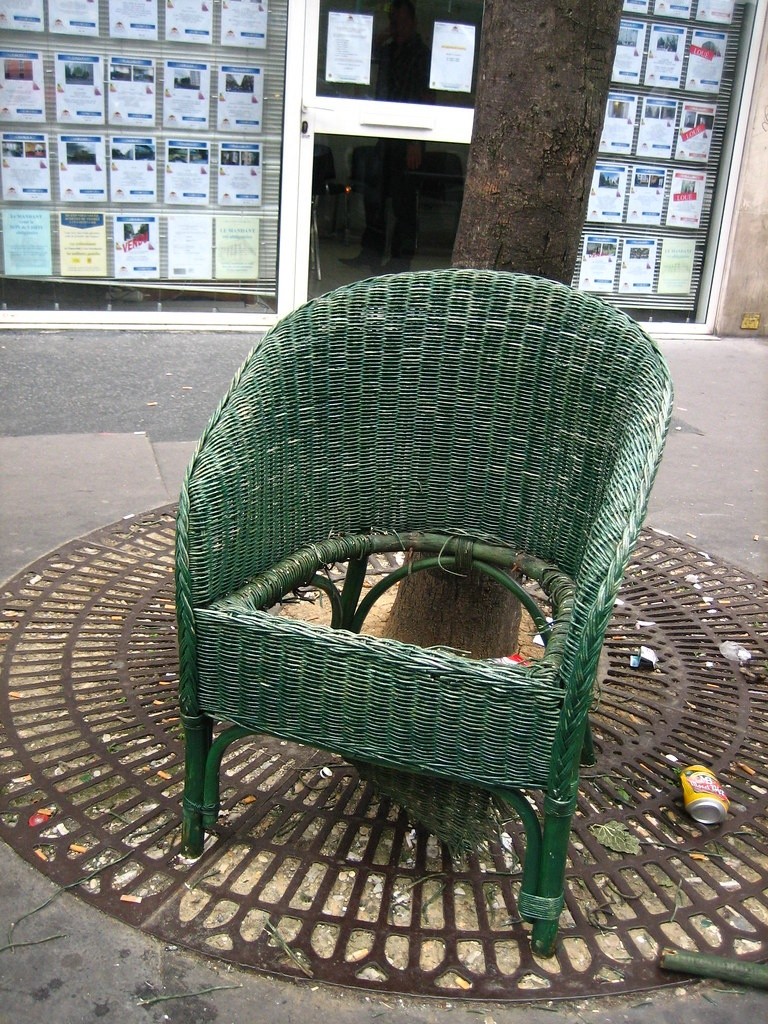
<box><xmin>337</xmin><ymin>0</ymin><xmax>438</xmax><ymax>277</ymax></box>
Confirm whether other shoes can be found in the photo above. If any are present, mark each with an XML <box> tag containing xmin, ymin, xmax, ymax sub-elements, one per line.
<box><xmin>390</xmin><ymin>255</ymin><xmax>411</xmax><ymax>274</ymax></box>
<box><xmin>338</xmin><ymin>251</ymin><xmax>383</xmax><ymax>268</ymax></box>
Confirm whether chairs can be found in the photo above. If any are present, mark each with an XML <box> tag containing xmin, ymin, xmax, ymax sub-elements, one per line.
<box><xmin>171</xmin><ymin>268</ymin><xmax>673</xmax><ymax>957</ymax></box>
<box><xmin>411</xmin><ymin>151</ymin><xmax>464</xmax><ymax>255</ymax></box>
<box><xmin>309</xmin><ymin>143</ymin><xmax>373</xmax><ymax>281</ymax></box>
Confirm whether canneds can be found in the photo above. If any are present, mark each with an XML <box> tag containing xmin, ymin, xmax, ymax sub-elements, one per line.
<box><xmin>680</xmin><ymin>765</ymin><xmax>730</xmax><ymax>824</ymax></box>
